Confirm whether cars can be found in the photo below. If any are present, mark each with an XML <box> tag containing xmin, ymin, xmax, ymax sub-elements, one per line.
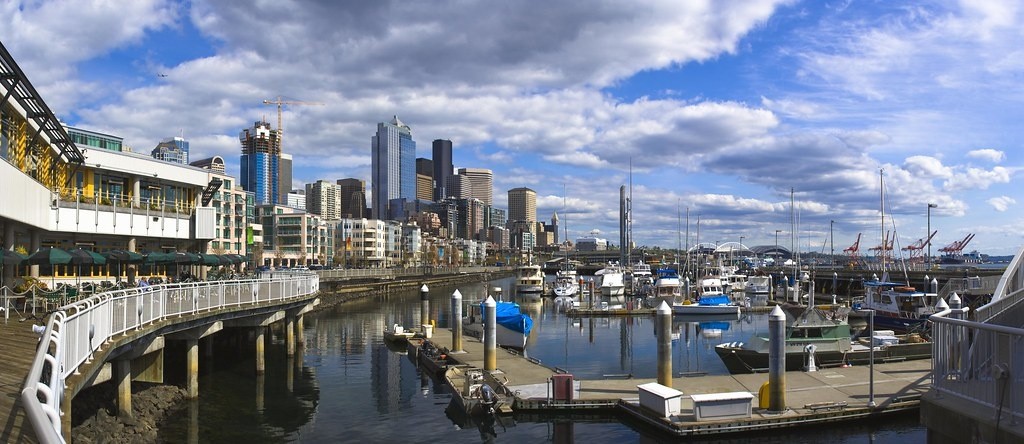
<box><xmin>254</xmin><ymin>261</ymin><xmax>364</xmax><ymax>276</ymax></box>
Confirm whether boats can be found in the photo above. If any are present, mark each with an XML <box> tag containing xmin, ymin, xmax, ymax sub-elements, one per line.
<box><xmin>382</xmin><ymin>322</ymin><xmax>416</xmax><ymax>342</ymax></box>
<box><xmin>514</xmin><ymin>248</ymin><xmax>548</xmax><ymax>292</ymax></box>
<box><xmin>445</xmin><ymin>363</ymin><xmax>517</xmax><ymax>415</ymax></box>
<box><xmin>461</xmin><ymin>284</ymin><xmax>534</xmax><ymax>353</ymax></box>
<box><xmin>405</xmin><ymin>336</ymin><xmax>451</xmax><ymax>376</ymax></box>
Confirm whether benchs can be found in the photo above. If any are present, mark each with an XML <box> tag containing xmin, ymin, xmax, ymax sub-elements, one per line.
<box><xmin>638</xmin><ymin>382</ymin><xmax>683</xmax><ymax>417</ymax></box>
<box><xmin>690</xmin><ymin>392</ymin><xmax>754</xmax><ymax>421</ymax></box>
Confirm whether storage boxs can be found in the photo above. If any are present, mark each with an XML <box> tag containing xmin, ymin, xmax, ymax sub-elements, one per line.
<box><xmin>872</xmin><ymin>336</ymin><xmax>899</xmax><ymax>348</ymax></box>
<box><xmin>873</xmin><ymin>330</ymin><xmax>894</xmax><ymax>336</ymax></box>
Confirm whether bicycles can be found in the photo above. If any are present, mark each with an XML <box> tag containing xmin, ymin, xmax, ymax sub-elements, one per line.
<box><xmin>19</xmin><ymin>308</ymin><xmax>67</xmax><ymax>386</ymax></box>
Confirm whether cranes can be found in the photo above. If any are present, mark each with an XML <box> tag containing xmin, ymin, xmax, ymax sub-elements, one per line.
<box><xmin>262</xmin><ymin>94</ymin><xmax>327</xmax><ymax>205</ymax></box>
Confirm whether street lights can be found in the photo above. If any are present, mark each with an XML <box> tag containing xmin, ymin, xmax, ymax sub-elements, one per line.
<box><xmin>715</xmin><ymin>241</ymin><xmax>720</xmax><ymax>263</ymax></box>
<box><xmin>927</xmin><ymin>203</ymin><xmax>937</xmax><ymax>271</ymax></box>
<box><xmin>830</xmin><ymin>220</ymin><xmax>838</xmax><ymax>271</ymax></box>
<box><xmin>739</xmin><ymin>236</ymin><xmax>746</xmax><ymax>268</ymax></box>
<box><xmin>775</xmin><ymin>229</ymin><xmax>783</xmax><ymax>268</ymax></box>
<box><xmin>224</xmin><ymin>214</ymin><xmax>255</xmax><ymax>256</ymax></box>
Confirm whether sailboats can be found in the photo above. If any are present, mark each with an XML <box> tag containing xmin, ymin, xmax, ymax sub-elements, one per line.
<box><xmin>550</xmin><ymin>156</ymin><xmax>939</xmax><ymax>374</ymax></box>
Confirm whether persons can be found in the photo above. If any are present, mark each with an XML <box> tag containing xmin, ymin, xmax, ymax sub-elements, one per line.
<box><xmin>139</xmin><ymin>277</ymin><xmax>149</xmax><ymax>292</ymax></box>
<box><xmin>167</xmin><ymin>276</ymin><xmax>174</xmax><ymax>288</ymax></box>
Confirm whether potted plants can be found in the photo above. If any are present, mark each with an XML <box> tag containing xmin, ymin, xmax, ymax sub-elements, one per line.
<box><xmin>15</xmin><ymin>276</ymin><xmax>47</xmax><ymax>298</ymax></box>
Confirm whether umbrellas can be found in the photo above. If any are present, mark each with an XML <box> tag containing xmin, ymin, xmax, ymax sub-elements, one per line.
<box><xmin>0</xmin><ymin>247</ymin><xmax>250</xmax><ymax>307</ymax></box>
